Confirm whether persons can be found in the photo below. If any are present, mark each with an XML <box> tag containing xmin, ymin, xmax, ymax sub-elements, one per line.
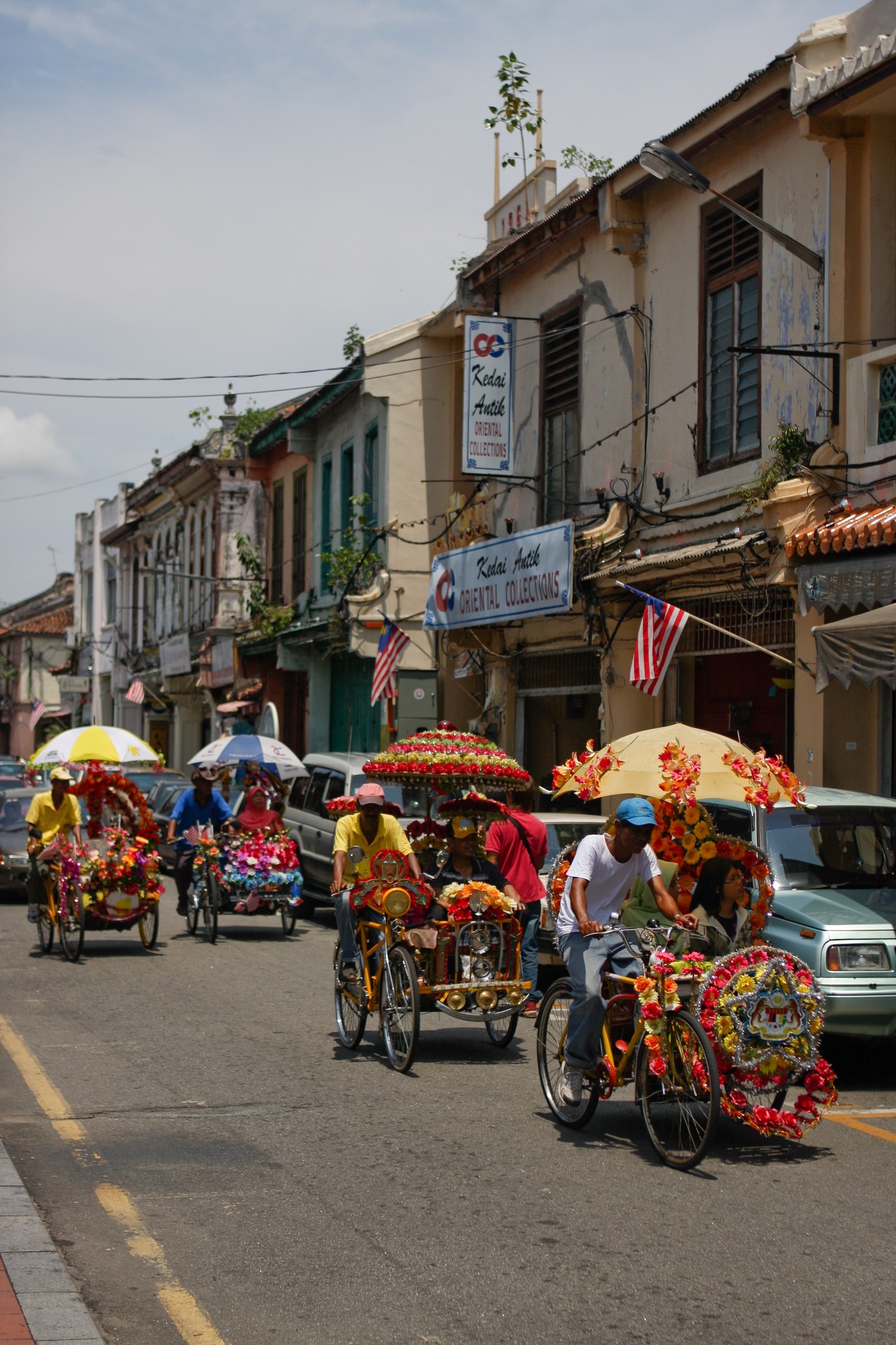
<box><xmin>26</xmin><ymin>768</ymin><xmax>85</xmax><ymax>922</ymax></box>
<box><xmin>554</xmin><ymin>796</ymin><xmax>700</xmax><ymax>1109</ymax></box>
<box><xmin>166</xmin><ymin>767</ymin><xmax>235</xmax><ymax>915</ymax></box>
<box><xmin>330</xmin><ymin>784</ymin><xmax>435</xmax><ymax>985</ymax></box>
<box><xmin>235</xmin><ymin>786</ymin><xmax>286</xmax><ymax>836</ymax></box>
<box><xmin>683</xmin><ymin>857</ymin><xmax>753</xmax><ymax>952</ymax></box>
<box><xmin>425</xmin><ymin>814</ymin><xmax>526</xmax><ymax>983</ymax></box>
<box><xmin>485</xmin><ymin>785</ymin><xmax>549</xmax><ymax>1019</ymax></box>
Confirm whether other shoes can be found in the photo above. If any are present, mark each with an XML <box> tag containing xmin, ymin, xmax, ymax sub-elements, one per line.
<box><xmin>63</xmin><ymin>915</ymin><xmax>81</xmax><ymax>931</ymax></box>
<box><xmin>176</xmin><ymin>903</ymin><xmax>188</xmax><ymax>916</ymax></box>
<box><xmin>27</xmin><ymin>902</ymin><xmax>41</xmax><ymax>923</ymax></box>
<box><xmin>518</xmin><ymin>1001</ymin><xmax>537</xmax><ymax>1018</ymax></box>
<box><xmin>338</xmin><ymin>964</ymin><xmax>359</xmax><ymax>984</ymax></box>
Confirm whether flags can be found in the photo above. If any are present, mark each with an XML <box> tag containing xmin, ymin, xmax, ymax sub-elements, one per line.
<box><xmin>370</xmin><ymin>609</ymin><xmax>413</xmax><ymax>708</ymax></box>
<box><xmin>28</xmin><ymin>697</ymin><xmax>46</xmax><ymax>727</ymax></box>
<box><xmin>125</xmin><ymin>670</ymin><xmax>146</xmax><ymax>704</ymax></box>
<box><xmin>614</xmin><ymin>579</ymin><xmax>690</xmax><ymax>697</ymax></box>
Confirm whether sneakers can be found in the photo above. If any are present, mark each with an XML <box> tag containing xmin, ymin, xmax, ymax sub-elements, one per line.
<box><xmin>634</xmin><ymin>1086</ymin><xmax>682</xmax><ymax>1104</ymax></box>
<box><xmin>558</xmin><ymin>1062</ymin><xmax>586</xmax><ymax>1105</ymax></box>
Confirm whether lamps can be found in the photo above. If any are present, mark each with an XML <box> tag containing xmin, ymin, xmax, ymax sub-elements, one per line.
<box><xmin>595</xmin><ymin>488</ymin><xmax>609</xmax><ymax>509</ymax></box>
<box><xmin>640</xmin><ymin>142</ymin><xmax>825</xmax><ymax>274</ymax></box>
<box><xmin>653</xmin><ymin>472</ymin><xmax>671</xmax><ymax>499</ymax></box>
<box><xmin>823</xmin><ymin>498</ymin><xmax>851</xmax><ymax>525</ymax></box>
<box><xmin>617</xmin><ymin>548</ymin><xmax>642</xmax><ymax>566</ymax></box>
<box><xmin>715</xmin><ymin>526</ymin><xmax>742</xmax><ymax>548</ymax></box>
<box><xmin>504</xmin><ymin>518</ymin><xmax>516</xmax><ymax>535</ymax></box>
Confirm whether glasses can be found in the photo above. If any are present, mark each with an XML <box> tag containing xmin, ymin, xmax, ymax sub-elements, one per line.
<box><xmin>724</xmin><ymin>873</ymin><xmax>745</xmax><ymax>884</ymax></box>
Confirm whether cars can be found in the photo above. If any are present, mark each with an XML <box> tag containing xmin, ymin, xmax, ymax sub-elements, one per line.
<box><xmin>689</xmin><ymin>785</ymin><xmax>896</xmax><ymax>1053</ymax></box>
<box><xmin>526</xmin><ymin>810</ymin><xmax>610</xmax><ymax>971</ymax></box>
<box><xmin>280</xmin><ymin>748</ymin><xmax>467</xmax><ymax>921</ymax></box>
<box><xmin>0</xmin><ymin>755</ymin><xmax>251</xmax><ymax>905</ymax></box>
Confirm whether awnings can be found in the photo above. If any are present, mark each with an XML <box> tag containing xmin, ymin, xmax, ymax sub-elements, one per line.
<box><xmin>811</xmin><ymin>601</ymin><xmax>896</xmax><ymax>692</ymax></box>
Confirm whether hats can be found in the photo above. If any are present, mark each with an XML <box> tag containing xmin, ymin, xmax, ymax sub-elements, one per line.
<box><xmin>616</xmin><ymin>798</ymin><xmax>659</xmax><ymax>827</ymax></box>
<box><xmin>356</xmin><ymin>783</ymin><xmax>385</xmax><ymax>805</ymax></box>
<box><xmin>50</xmin><ymin>767</ymin><xmax>74</xmax><ymax>781</ymax></box>
<box><xmin>191</xmin><ymin>768</ymin><xmax>219</xmax><ymax>781</ymax></box>
<box><xmin>445</xmin><ymin>816</ymin><xmax>478</xmax><ymax>840</ymax></box>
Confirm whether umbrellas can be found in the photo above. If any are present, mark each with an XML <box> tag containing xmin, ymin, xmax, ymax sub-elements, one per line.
<box><xmin>186</xmin><ymin>729</ymin><xmax>311</xmax><ymax>784</ymax></box>
<box><xmin>552</xmin><ymin>723</ymin><xmax>794</xmax><ymax>807</ymax></box>
<box><xmin>29</xmin><ymin>723</ymin><xmax>159</xmax><ymax>765</ymax></box>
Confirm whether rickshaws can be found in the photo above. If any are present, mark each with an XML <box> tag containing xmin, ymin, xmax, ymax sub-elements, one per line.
<box><xmin>327</xmin><ymin>729</ymin><xmax>538</xmax><ymax>1074</ymax></box>
<box><xmin>20</xmin><ymin>723</ymin><xmax>167</xmax><ymax>962</ymax></box>
<box><xmin>167</xmin><ymin>733</ymin><xmax>312</xmax><ymax>943</ymax></box>
<box><xmin>533</xmin><ymin>723</ymin><xmax>825</xmax><ymax>1171</ymax></box>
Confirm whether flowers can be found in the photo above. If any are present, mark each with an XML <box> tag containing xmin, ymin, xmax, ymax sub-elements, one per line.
<box><xmin>548</xmin><ymin>738</ymin><xmax>839</xmax><ymax>1140</ymax></box>
<box><xmin>22</xmin><ymin>760</ymin><xmax>166</xmax><ymax>919</ymax></box>
<box><xmin>327</xmin><ymin>729</ymin><xmax>531</xmax><ymax>911</ymax></box>
<box><xmin>192</xmin><ymin>825</ymin><xmax>303</xmax><ymax>891</ymax></box>
